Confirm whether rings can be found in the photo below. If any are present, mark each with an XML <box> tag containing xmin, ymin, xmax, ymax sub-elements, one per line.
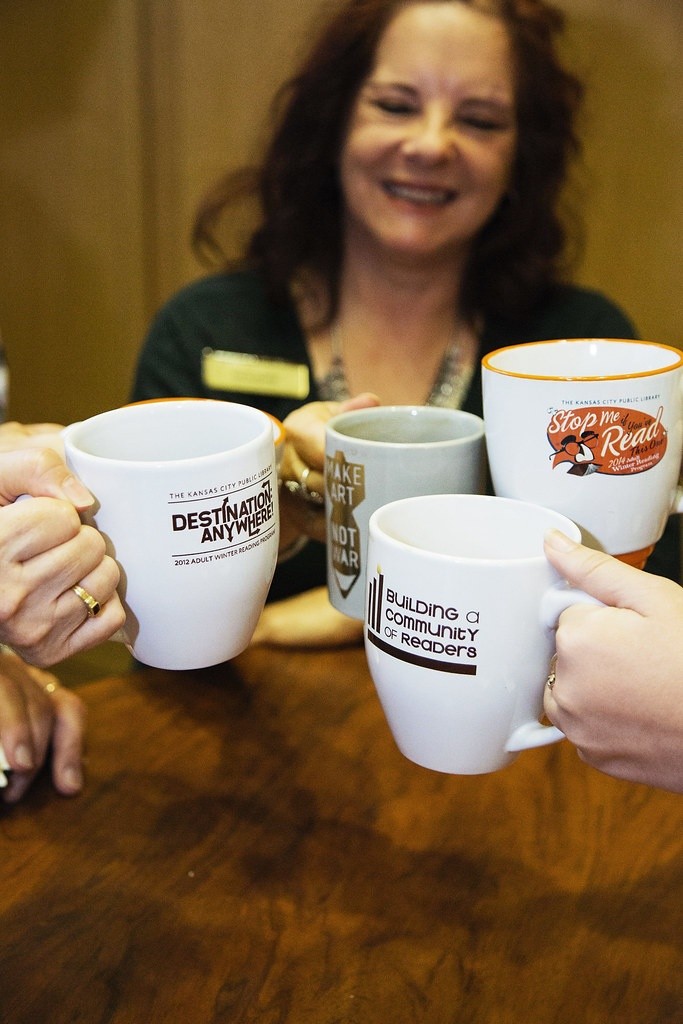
<box><xmin>73</xmin><ymin>586</ymin><xmax>103</xmax><ymax>620</ymax></box>
<box><xmin>547</xmin><ymin>654</ymin><xmax>559</xmax><ymax>689</ymax></box>
<box><xmin>42</xmin><ymin>680</ymin><xmax>62</xmax><ymax>696</ymax></box>
<box><xmin>285</xmin><ymin>465</ymin><xmax>322</xmax><ymax>503</ymax></box>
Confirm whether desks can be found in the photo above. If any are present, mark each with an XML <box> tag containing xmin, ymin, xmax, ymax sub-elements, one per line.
<box><xmin>0</xmin><ymin>640</ymin><xmax>683</xmax><ymax>1024</ymax></box>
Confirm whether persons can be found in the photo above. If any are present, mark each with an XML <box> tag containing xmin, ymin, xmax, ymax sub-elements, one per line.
<box><xmin>0</xmin><ymin>445</ymin><xmax>126</xmax><ymax>807</ymax></box>
<box><xmin>125</xmin><ymin>1</ymin><xmax>683</xmax><ymax>646</ymax></box>
<box><xmin>539</xmin><ymin>530</ymin><xmax>683</xmax><ymax>792</ymax></box>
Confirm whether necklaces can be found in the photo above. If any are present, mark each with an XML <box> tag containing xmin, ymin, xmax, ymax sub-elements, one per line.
<box><xmin>322</xmin><ymin>319</ymin><xmax>470</xmax><ymax>409</ymax></box>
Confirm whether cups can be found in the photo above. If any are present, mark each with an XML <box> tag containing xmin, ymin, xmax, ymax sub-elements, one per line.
<box><xmin>121</xmin><ymin>398</ymin><xmax>285</xmax><ymax>489</ymax></box>
<box><xmin>15</xmin><ymin>400</ymin><xmax>280</xmax><ymax>671</ymax></box>
<box><xmin>365</xmin><ymin>494</ymin><xmax>608</xmax><ymax>775</ymax></box>
<box><xmin>481</xmin><ymin>338</ymin><xmax>683</xmax><ymax>570</ymax></box>
<box><xmin>324</xmin><ymin>407</ymin><xmax>484</xmax><ymax>619</ymax></box>
